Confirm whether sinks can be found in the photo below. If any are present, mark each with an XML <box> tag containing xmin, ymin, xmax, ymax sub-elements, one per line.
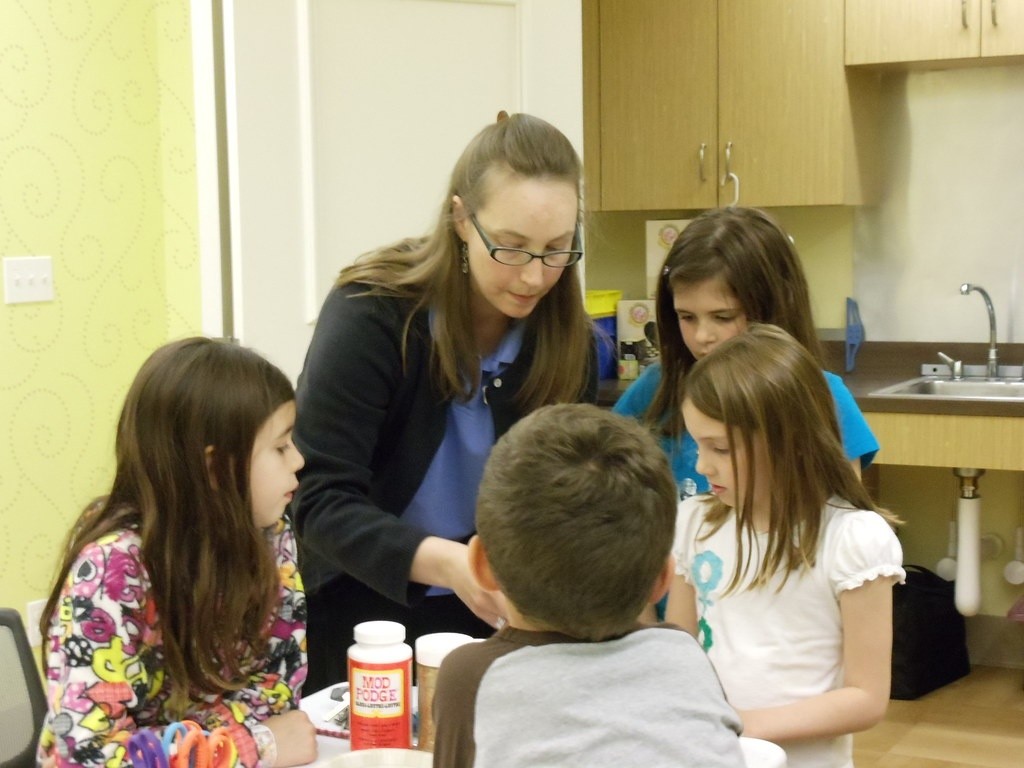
<box><xmin>867</xmin><ymin>375</ymin><xmax>1024</xmax><ymax>401</ymax></box>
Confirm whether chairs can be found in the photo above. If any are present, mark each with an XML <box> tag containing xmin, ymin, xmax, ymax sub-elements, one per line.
<box><xmin>0</xmin><ymin>607</ymin><xmax>48</xmax><ymax>768</ymax></box>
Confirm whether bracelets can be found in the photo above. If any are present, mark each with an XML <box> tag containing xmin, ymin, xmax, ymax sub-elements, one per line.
<box><xmin>248</xmin><ymin>725</ymin><xmax>276</xmax><ymax>768</ymax></box>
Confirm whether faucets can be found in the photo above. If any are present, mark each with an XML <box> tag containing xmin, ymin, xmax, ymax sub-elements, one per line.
<box><xmin>960</xmin><ymin>282</ymin><xmax>1000</xmax><ymax>377</ymax></box>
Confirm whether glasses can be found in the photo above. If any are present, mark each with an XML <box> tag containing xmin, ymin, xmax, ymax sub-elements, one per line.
<box><xmin>465</xmin><ymin>203</ymin><xmax>582</xmax><ymax>268</ymax></box>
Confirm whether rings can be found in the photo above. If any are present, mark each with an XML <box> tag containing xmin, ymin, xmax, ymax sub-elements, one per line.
<box><xmin>495</xmin><ymin>617</ymin><xmax>506</xmax><ymax>629</ymax></box>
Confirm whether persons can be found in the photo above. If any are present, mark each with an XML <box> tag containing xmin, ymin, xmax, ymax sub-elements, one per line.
<box><xmin>287</xmin><ymin>110</ymin><xmax>599</xmax><ymax>700</ymax></box>
<box><xmin>37</xmin><ymin>337</ymin><xmax>316</xmax><ymax>768</ymax></box>
<box><xmin>664</xmin><ymin>325</ymin><xmax>905</xmax><ymax>767</ymax></box>
<box><xmin>609</xmin><ymin>206</ymin><xmax>879</xmax><ymax>625</ymax></box>
<box><xmin>433</xmin><ymin>407</ymin><xmax>747</xmax><ymax>767</ymax></box>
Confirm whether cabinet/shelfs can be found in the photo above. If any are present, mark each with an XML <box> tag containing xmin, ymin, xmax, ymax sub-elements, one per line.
<box><xmin>580</xmin><ymin>0</ymin><xmax>1024</xmax><ymax>211</ymax></box>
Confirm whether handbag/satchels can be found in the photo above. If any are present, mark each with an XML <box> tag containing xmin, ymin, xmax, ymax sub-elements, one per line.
<box><xmin>890</xmin><ymin>564</ymin><xmax>972</xmax><ymax>700</ymax></box>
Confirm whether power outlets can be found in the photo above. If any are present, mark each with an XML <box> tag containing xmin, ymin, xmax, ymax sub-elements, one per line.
<box><xmin>27</xmin><ymin>600</ymin><xmax>48</xmax><ymax>646</ymax></box>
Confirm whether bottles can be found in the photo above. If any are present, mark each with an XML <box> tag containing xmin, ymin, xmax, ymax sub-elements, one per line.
<box><xmin>346</xmin><ymin>621</ymin><xmax>414</xmax><ymax>750</ymax></box>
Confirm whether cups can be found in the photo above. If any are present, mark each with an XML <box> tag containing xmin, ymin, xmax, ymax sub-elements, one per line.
<box><xmin>414</xmin><ymin>632</ymin><xmax>473</xmax><ymax>753</ymax></box>
